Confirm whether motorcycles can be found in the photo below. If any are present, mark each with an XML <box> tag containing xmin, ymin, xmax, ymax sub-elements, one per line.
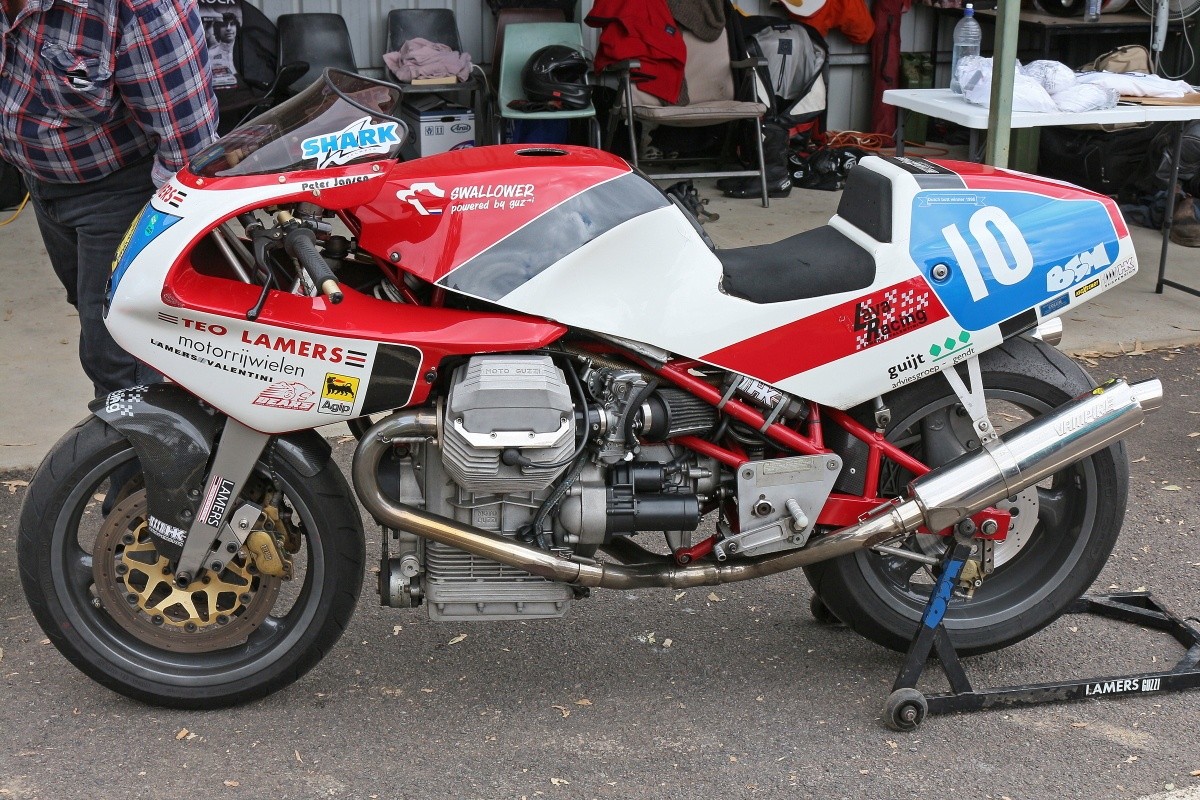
<box><xmin>14</xmin><ymin>61</ymin><xmax>1165</xmax><ymax>710</ymax></box>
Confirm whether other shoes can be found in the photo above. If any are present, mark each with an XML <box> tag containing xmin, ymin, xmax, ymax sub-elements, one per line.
<box><xmin>1170</xmin><ymin>192</ymin><xmax>1200</xmax><ymax>247</ymax></box>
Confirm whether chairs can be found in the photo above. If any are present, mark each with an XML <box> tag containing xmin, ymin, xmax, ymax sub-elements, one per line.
<box><xmin>603</xmin><ymin>27</ymin><xmax>770</xmax><ymax>208</ymax></box>
<box><xmin>275</xmin><ymin>12</ymin><xmax>359</xmax><ymax>105</ymax></box>
<box><xmin>383</xmin><ymin>8</ymin><xmax>495</xmax><ymax>147</ymax></box>
<box><xmin>482</xmin><ymin>7</ymin><xmax>601</xmax><ymax>151</ymax></box>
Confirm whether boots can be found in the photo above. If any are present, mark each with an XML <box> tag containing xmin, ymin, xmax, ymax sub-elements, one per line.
<box><xmin>718</xmin><ymin>123</ymin><xmax>792</xmax><ymax>198</ymax></box>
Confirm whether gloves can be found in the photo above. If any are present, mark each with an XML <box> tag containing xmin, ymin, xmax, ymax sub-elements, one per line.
<box><xmin>508</xmin><ymin>100</ymin><xmax>553</xmax><ymax>112</ymax></box>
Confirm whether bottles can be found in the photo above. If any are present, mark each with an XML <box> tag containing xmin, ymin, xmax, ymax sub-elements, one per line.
<box><xmin>1084</xmin><ymin>0</ymin><xmax>1101</xmax><ymax>21</ymax></box>
<box><xmin>950</xmin><ymin>4</ymin><xmax>982</xmax><ymax>98</ymax></box>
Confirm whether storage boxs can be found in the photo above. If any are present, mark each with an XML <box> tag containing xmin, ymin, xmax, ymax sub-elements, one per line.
<box><xmin>400</xmin><ymin>95</ymin><xmax>476</xmax><ymax>162</ymax></box>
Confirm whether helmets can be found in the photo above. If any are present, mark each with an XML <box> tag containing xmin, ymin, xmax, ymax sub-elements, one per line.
<box><xmin>524</xmin><ymin>45</ymin><xmax>591</xmax><ymax>105</ymax></box>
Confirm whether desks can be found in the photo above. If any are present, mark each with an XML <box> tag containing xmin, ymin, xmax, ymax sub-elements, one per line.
<box><xmin>930</xmin><ymin>7</ymin><xmax>1196</xmax><ymax>80</ymax></box>
<box><xmin>883</xmin><ymin>88</ymin><xmax>1199</xmax><ymax>296</ymax></box>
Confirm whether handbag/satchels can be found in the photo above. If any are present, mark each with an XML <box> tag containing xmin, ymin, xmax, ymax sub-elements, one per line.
<box><xmin>1083</xmin><ymin>44</ymin><xmax>1154</xmax><ymax>74</ymax></box>
<box><xmin>1037</xmin><ymin>119</ymin><xmax>1200</xmax><ymax>228</ymax></box>
<box><xmin>789</xmin><ymin>146</ymin><xmax>860</xmax><ymax>190</ymax></box>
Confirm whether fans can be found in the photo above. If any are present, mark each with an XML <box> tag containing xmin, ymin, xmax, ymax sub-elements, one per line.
<box><xmin>1136</xmin><ymin>0</ymin><xmax>1200</xmax><ymax>75</ymax></box>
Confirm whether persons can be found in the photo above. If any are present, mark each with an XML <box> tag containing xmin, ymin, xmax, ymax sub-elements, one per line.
<box><xmin>201</xmin><ymin>13</ymin><xmax>239</xmax><ymax>77</ymax></box>
<box><xmin>0</xmin><ymin>0</ymin><xmax>221</xmax><ymax>553</ymax></box>
